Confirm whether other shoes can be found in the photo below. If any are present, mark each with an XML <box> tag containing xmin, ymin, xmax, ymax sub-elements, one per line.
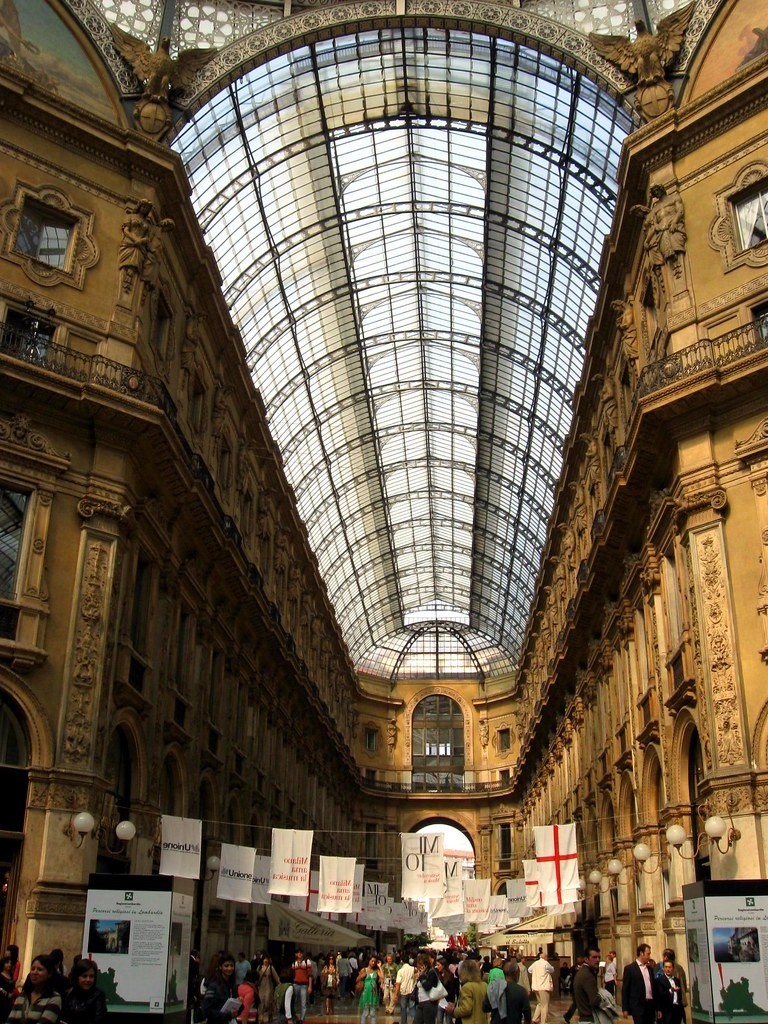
<box><xmin>563</xmin><ymin>1015</ymin><xmax>570</xmax><ymax>1024</ymax></box>
<box><xmin>531</xmin><ymin>1021</ymin><xmax>537</xmax><ymax>1024</ymax></box>
<box><xmin>326</xmin><ymin>1007</ymin><xmax>334</xmax><ymax>1015</ymax></box>
<box><xmin>385</xmin><ymin>1010</ymin><xmax>394</xmax><ymax>1015</ymax></box>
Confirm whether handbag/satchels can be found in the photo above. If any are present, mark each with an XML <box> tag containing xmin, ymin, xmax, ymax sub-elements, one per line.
<box><xmin>355</xmin><ymin>968</ymin><xmax>365</xmax><ymax>991</ymax></box>
<box><xmin>353</xmin><ymin>969</ymin><xmax>359</xmax><ymax>980</ymax></box>
<box><xmin>390</xmin><ymin>980</ymin><xmax>394</xmax><ymax>990</ymax></box>
<box><xmin>427</xmin><ymin>969</ymin><xmax>448</xmax><ymax>1001</ymax></box>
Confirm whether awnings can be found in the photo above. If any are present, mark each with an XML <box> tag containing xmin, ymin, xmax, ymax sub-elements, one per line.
<box><xmin>265</xmin><ymin>897</ymin><xmax>373</xmax><ymax>945</ymax></box>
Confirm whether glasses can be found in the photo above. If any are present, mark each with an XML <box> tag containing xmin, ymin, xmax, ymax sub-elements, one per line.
<box><xmin>330</xmin><ymin>958</ymin><xmax>334</xmax><ymax>960</ymax></box>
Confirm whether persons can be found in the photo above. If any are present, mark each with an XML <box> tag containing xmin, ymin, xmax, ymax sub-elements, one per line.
<box><xmin>648</xmin><ymin>181</ymin><xmax>687</xmax><ymax>259</ymax></box>
<box><xmin>478</xmin><ymin>719</ymin><xmax>489</xmax><ymax>746</ymax></box>
<box><xmin>628</xmin><ymin>203</ymin><xmax>666</xmax><ymax>271</ymax></box>
<box><xmin>0</xmin><ymin>943</ymin><xmax>688</xmax><ymax>1024</ymax></box>
<box><xmin>514</xmin><ymin>300</ymin><xmax>640</xmax><ymax>740</ymax></box>
<box><xmin>116</xmin><ymin>198</ymin><xmax>155</xmax><ymax>289</ymax></box>
<box><xmin>181</xmin><ymin>309</ymin><xmax>362</xmax><ymax>742</ymax></box>
<box><xmin>387</xmin><ymin>719</ymin><xmax>397</xmax><ymax>753</ymax></box>
<box><xmin>142</xmin><ymin>215</ymin><xmax>177</xmax><ymax>288</ymax></box>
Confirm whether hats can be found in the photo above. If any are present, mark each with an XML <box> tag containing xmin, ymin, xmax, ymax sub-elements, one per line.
<box><xmin>295</xmin><ymin>946</ymin><xmax>302</xmax><ymax>952</ymax></box>
<box><xmin>438</xmin><ymin>958</ymin><xmax>448</xmax><ymax>966</ymax></box>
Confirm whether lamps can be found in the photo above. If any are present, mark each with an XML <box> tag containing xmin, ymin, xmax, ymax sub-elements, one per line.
<box><xmin>95</xmin><ymin>821</ymin><xmax>136</xmax><ymax>855</ymax></box>
<box><xmin>62</xmin><ymin>789</ymin><xmax>94</xmax><ymax>849</ymax></box>
<box><xmin>705</xmin><ymin>800</ymin><xmax>740</xmax><ymax>855</ymax></box>
<box><xmin>194</xmin><ymin>855</ymin><xmax>220</xmax><ymax>882</ymax></box>
<box><xmin>578</xmin><ymin>828</ymin><xmax>667</xmax><ymax>897</ymax></box>
<box><xmin>666</xmin><ymin>824</ymin><xmax>707</xmax><ymax>859</ymax></box>
